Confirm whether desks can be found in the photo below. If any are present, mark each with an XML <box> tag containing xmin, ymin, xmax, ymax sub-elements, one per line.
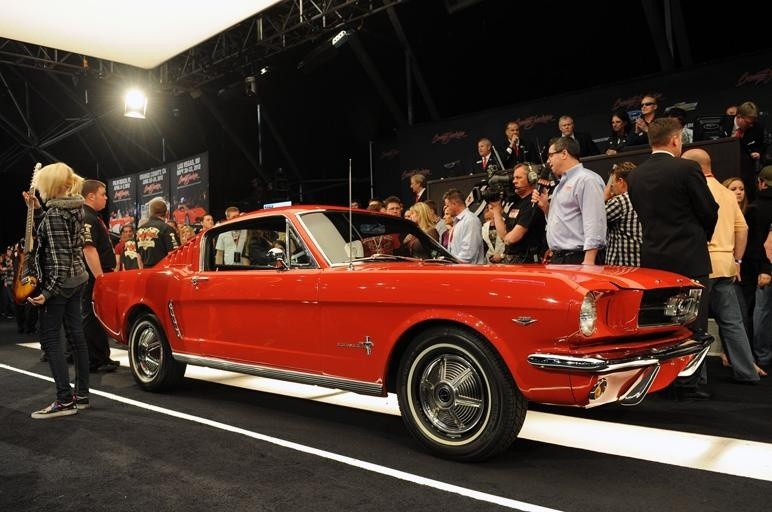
<box><xmin>426</xmin><ymin>137</ymin><xmax>743</xmax><ymax>219</ymax></box>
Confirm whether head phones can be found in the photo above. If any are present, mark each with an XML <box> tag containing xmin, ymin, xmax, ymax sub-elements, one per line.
<box><xmin>524</xmin><ymin>161</ymin><xmax>538</xmax><ymax>184</ymax></box>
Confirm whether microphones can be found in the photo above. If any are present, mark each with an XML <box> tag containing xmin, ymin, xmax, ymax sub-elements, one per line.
<box><xmin>515</xmin><ymin>185</ymin><xmax>531</xmax><ymax>189</ymax></box>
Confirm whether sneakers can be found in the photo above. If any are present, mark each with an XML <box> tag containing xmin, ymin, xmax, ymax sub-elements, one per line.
<box><xmin>31</xmin><ymin>396</ymin><xmax>90</xmax><ymax>418</ymax></box>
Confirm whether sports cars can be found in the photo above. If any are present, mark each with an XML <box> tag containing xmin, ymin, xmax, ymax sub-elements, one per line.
<box><xmin>92</xmin><ymin>203</ymin><xmax>718</xmax><ymax>464</ymax></box>
<box><xmin>172</xmin><ymin>203</ymin><xmax>207</xmax><ymax>225</ymax></box>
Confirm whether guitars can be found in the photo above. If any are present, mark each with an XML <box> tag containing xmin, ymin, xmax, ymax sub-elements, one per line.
<box><xmin>13</xmin><ymin>163</ymin><xmax>42</xmax><ymax>305</ymax></box>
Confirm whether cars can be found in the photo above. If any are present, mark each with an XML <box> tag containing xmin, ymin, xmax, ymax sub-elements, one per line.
<box><xmin>108</xmin><ymin>213</ymin><xmax>134</xmax><ymax>232</ymax></box>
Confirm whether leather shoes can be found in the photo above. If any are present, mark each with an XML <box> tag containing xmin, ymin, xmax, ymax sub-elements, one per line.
<box><xmin>658</xmin><ymin>385</ymin><xmax>713</xmax><ymax>402</ymax></box>
<box><xmin>90</xmin><ymin>361</ymin><xmax>120</xmax><ymax>373</ymax></box>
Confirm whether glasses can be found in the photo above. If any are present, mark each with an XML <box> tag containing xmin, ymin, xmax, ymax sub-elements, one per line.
<box><xmin>641</xmin><ymin>103</ymin><xmax>656</xmax><ymax>107</ymax></box>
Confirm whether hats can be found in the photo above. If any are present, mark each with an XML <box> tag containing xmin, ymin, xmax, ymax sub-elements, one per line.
<box><xmin>759</xmin><ymin>165</ymin><xmax>772</xmax><ymax>187</ymax></box>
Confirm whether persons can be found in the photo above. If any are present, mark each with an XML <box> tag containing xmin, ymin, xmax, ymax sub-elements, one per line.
<box><xmin>0</xmin><ymin>234</ymin><xmax>77</xmax><ymax>364</ymax></box>
<box><xmin>21</xmin><ymin>161</ymin><xmax>91</xmax><ymax>419</ymax></box>
<box><xmin>79</xmin><ymin>179</ymin><xmax>121</xmax><ymax>374</ymax></box>
<box><xmin>107</xmin><ymin>95</ymin><xmax>772</xmax><ymax>400</ymax></box>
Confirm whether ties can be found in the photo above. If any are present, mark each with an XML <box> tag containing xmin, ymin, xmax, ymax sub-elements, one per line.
<box><xmin>737</xmin><ymin>128</ymin><xmax>743</xmax><ymax>137</ymax></box>
<box><xmin>483</xmin><ymin>158</ymin><xmax>486</xmax><ymax>170</ymax></box>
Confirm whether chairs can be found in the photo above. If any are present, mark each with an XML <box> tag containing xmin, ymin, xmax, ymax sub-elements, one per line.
<box><xmin>248</xmin><ymin>236</ymin><xmax>277</xmax><ymax>266</ymax></box>
